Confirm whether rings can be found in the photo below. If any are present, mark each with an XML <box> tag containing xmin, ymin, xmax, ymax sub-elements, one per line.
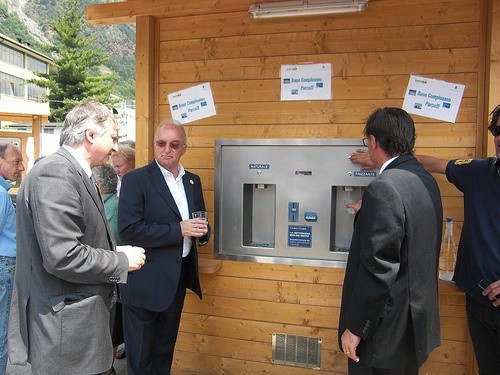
<box><xmin>346</xmin><ymin>351</ymin><xmax>350</xmax><ymax>354</ymax></box>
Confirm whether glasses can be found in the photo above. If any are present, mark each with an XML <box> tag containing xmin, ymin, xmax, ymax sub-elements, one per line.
<box><xmin>362</xmin><ymin>136</ymin><xmax>369</xmax><ymax>146</ymax></box>
<box><xmin>488</xmin><ymin>124</ymin><xmax>499</xmax><ymax>136</ymax></box>
<box><xmin>152</xmin><ymin>139</ymin><xmax>186</xmax><ymax>150</ymax></box>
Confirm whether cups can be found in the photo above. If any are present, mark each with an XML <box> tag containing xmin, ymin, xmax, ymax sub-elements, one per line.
<box><xmin>192</xmin><ymin>212</ymin><xmax>207</xmax><ymax>229</ymax></box>
<box><xmin>478</xmin><ymin>278</ymin><xmax>500</xmax><ymax>300</ymax></box>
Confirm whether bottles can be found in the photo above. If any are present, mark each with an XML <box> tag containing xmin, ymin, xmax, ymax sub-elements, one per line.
<box><xmin>438</xmin><ymin>217</ymin><xmax>457</xmax><ymax>281</ymax></box>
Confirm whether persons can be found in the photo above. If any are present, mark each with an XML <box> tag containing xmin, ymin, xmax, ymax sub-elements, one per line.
<box><xmin>350</xmin><ymin>104</ymin><xmax>500</xmax><ymax>375</ymax></box>
<box><xmin>0</xmin><ymin>143</ymin><xmax>25</xmax><ymax>375</ymax></box>
<box><xmin>338</xmin><ymin>106</ymin><xmax>443</xmax><ymax>375</ymax></box>
<box><xmin>118</xmin><ymin>119</ymin><xmax>210</xmax><ymax>375</ymax></box>
<box><xmin>91</xmin><ymin>140</ymin><xmax>135</xmax><ymax>359</ymax></box>
<box><xmin>5</xmin><ymin>101</ymin><xmax>145</xmax><ymax>375</ymax></box>
